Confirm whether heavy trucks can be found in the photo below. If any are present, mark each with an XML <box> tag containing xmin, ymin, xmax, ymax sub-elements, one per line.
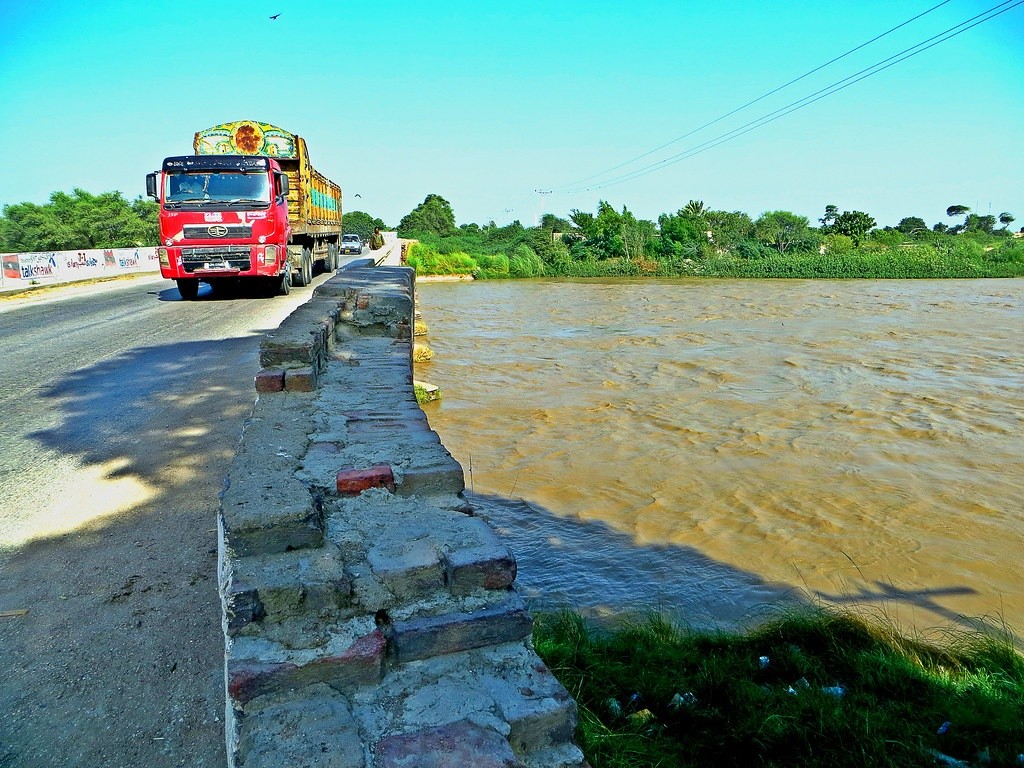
<box><xmin>146</xmin><ymin>115</ymin><xmax>344</xmax><ymax>298</ymax></box>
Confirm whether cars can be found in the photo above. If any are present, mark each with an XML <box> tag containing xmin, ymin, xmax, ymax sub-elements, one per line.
<box><xmin>339</xmin><ymin>234</ymin><xmax>363</xmax><ymax>254</ymax></box>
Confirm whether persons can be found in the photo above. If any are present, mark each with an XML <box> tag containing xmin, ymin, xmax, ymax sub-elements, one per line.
<box><xmin>179</xmin><ymin>176</ymin><xmax>203</xmax><ymax>194</ymax></box>
<box><xmin>369</xmin><ymin>227</ymin><xmax>385</xmax><ymax>250</ymax></box>
